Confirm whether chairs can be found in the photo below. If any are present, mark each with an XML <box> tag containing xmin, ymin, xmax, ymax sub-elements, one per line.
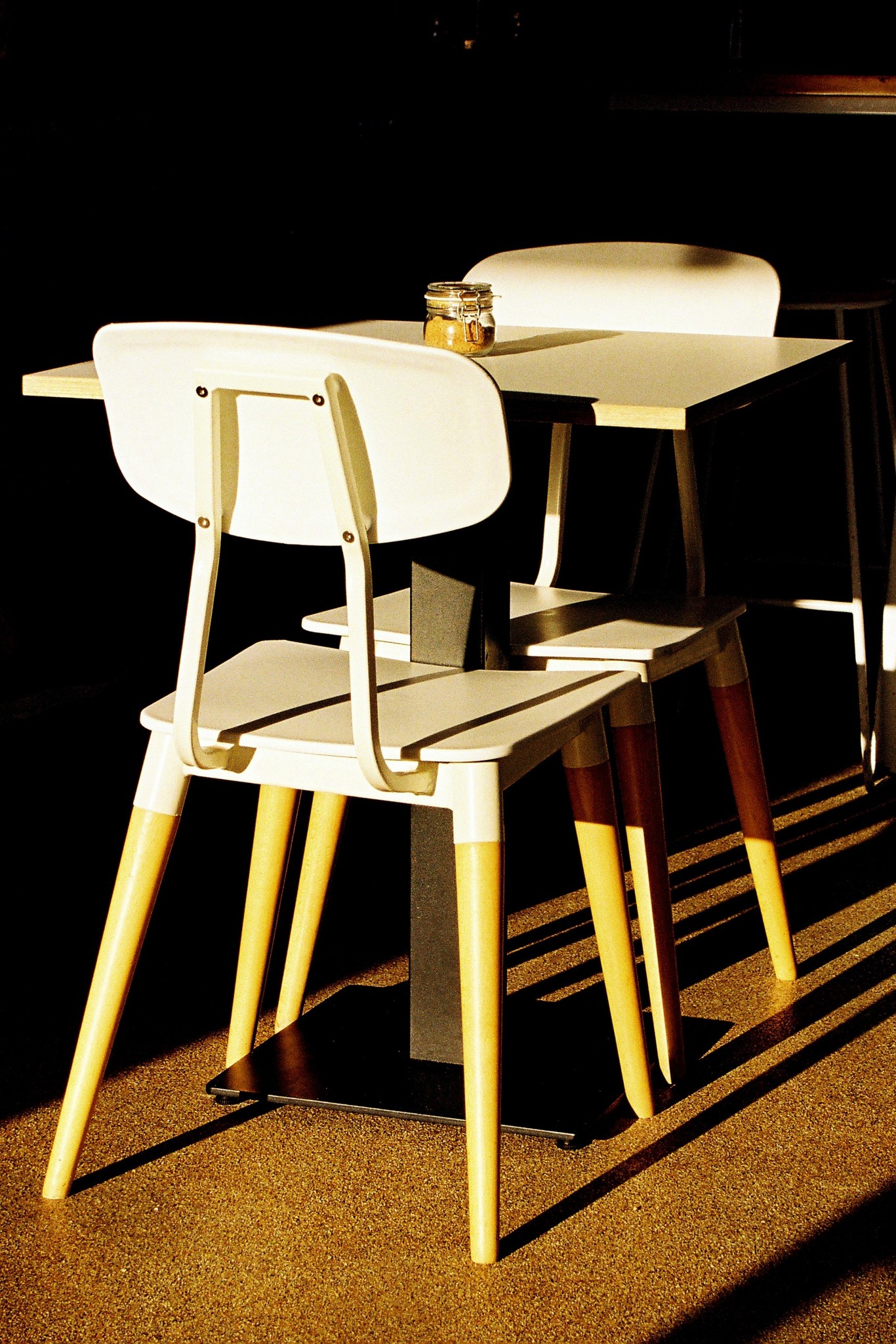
<box><xmin>268</xmin><ymin>240</ymin><xmax>799</xmax><ymax>1090</ymax></box>
<box><xmin>38</xmin><ymin>322</ymin><xmax>657</xmax><ymax>1265</ymax></box>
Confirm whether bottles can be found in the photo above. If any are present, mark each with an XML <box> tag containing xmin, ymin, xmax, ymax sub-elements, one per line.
<box><xmin>423</xmin><ymin>281</ymin><xmax>502</xmax><ymax>357</ymax></box>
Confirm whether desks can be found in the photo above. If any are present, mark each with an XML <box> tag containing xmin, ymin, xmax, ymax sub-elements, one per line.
<box><xmin>19</xmin><ymin>315</ymin><xmax>856</xmax><ymax>1145</ymax></box>
